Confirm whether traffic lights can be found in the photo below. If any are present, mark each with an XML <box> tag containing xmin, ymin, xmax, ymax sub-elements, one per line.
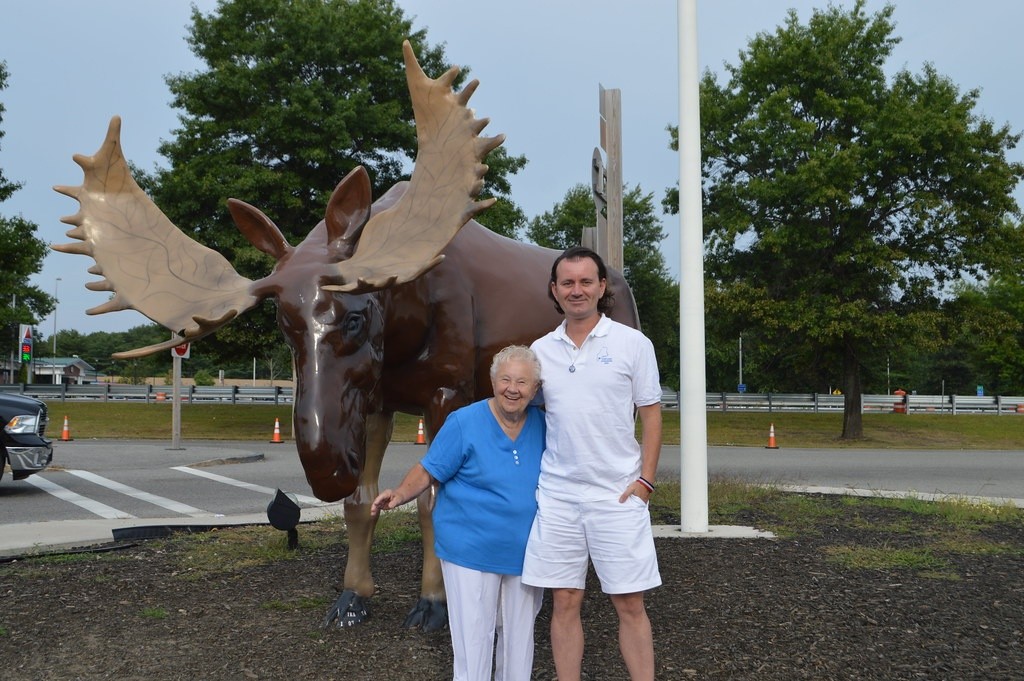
<box><xmin>22</xmin><ymin>342</ymin><xmax>31</xmax><ymax>365</ymax></box>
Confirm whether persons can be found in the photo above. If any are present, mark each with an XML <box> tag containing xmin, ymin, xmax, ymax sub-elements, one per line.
<box><xmin>521</xmin><ymin>246</ymin><xmax>662</xmax><ymax>680</ymax></box>
<box><xmin>371</xmin><ymin>344</ymin><xmax>546</xmax><ymax>681</ymax></box>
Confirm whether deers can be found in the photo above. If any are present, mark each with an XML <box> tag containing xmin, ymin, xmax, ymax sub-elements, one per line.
<box><xmin>48</xmin><ymin>39</ymin><xmax>641</xmax><ymax>634</ymax></box>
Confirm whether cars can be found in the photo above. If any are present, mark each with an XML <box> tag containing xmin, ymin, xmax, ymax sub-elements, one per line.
<box><xmin>661</xmin><ymin>384</ymin><xmax>678</xmax><ymax>408</ymax></box>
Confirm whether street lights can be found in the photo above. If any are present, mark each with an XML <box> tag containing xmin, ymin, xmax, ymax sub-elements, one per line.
<box><xmin>52</xmin><ymin>278</ymin><xmax>62</xmax><ymax>385</ymax></box>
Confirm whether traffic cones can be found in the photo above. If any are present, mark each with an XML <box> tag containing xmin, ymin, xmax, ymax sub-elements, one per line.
<box><xmin>765</xmin><ymin>423</ymin><xmax>779</xmax><ymax>449</ymax></box>
<box><xmin>413</xmin><ymin>418</ymin><xmax>426</xmax><ymax>444</ymax></box>
<box><xmin>269</xmin><ymin>417</ymin><xmax>284</xmax><ymax>443</ymax></box>
<box><xmin>57</xmin><ymin>415</ymin><xmax>74</xmax><ymax>441</ymax></box>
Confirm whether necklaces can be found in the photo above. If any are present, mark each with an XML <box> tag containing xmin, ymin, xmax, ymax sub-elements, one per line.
<box><xmin>495</xmin><ymin>407</ymin><xmax>520</xmax><ymax>429</ymax></box>
<box><xmin>563</xmin><ymin>340</ymin><xmax>580</xmax><ymax>373</ymax></box>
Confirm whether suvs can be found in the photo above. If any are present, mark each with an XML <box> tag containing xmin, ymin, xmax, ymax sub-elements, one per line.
<box><xmin>0</xmin><ymin>393</ymin><xmax>54</xmax><ymax>483</ymax></box>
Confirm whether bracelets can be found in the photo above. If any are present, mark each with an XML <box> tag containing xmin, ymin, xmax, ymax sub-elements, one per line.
<box><xmin>638</xmin><ymin>477</ymin><xmax>654</xmax><ymax>492</ymax></box>
